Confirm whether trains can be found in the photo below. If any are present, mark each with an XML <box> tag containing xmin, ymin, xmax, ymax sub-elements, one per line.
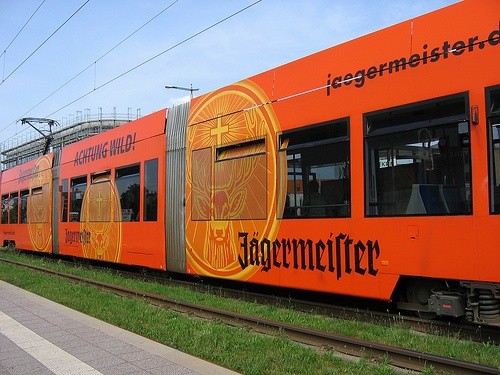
<box><xmin>0</xmin><ymin>0</ymin><xmax>500</xmax><ymax>338</ymax></box>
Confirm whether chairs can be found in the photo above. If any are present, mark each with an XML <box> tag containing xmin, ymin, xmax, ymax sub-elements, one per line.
<box><xmin>280</xmin><ymin>151</ymin><xmax>470</xmax><ymax>216</ymax></box>
